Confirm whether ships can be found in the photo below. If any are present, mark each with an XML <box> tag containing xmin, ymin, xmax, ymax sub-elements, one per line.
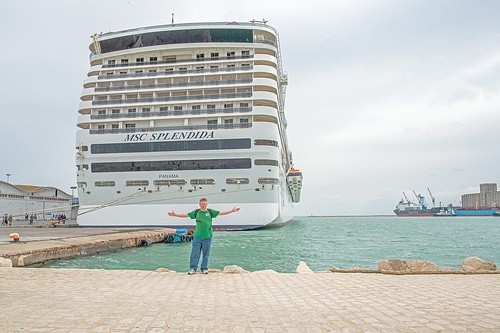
<box><xmin>75</xmin><ymin>10</ymin><xmax>304</xmax><ymax>232</ymax></box>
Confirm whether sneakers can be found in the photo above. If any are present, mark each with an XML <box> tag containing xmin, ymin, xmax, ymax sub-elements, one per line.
<box><xmin>188</xmin><ymin>269</ymin><xmax>196</xmax><ymax>274</ymax></box>
<box><xmin>203</xmin><ymin>269</ymin><xmax>209</xmax><ymax>274</ymax></box>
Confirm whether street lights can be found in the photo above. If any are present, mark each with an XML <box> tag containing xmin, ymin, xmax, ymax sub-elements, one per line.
<box><xmin>6</xmin><ymin>173</ymin><xmax>11</xmax><ymax>182</ymax></box>
<box><xmin>70</xmin><ymin>186</ymin><xmax>77</xmax><ymax>220</ymax></box>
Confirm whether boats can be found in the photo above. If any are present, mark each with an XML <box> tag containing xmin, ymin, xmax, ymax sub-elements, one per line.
<box><xmin>393</xmin><ymin>187</ymin><xmax>460</xmax><ymax>216</ymax></box>
<box><xmin>286</xmin><ymin>169</ymin><xmax>303</xmax><ymax>184</ymax></box>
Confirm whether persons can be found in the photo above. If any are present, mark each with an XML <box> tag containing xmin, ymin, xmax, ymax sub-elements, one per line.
<box><xmin>168</xmin><ymin>198</ymin><xmax>240</xmax><ymax>275</ymax></box>
<box><xmin>24</xmin><ymin>213</ymin><xmax>37</xmax><ymax>224</ymax></box>
<box><xmin>51</xmin><ymin>213</ymin><xmax>66</xmax><ymax>224</ymax></box>
<box><xmin>3</xmin><ymin>213</ymin><xmax>13</xmax><ymax>226</ymax></box>
